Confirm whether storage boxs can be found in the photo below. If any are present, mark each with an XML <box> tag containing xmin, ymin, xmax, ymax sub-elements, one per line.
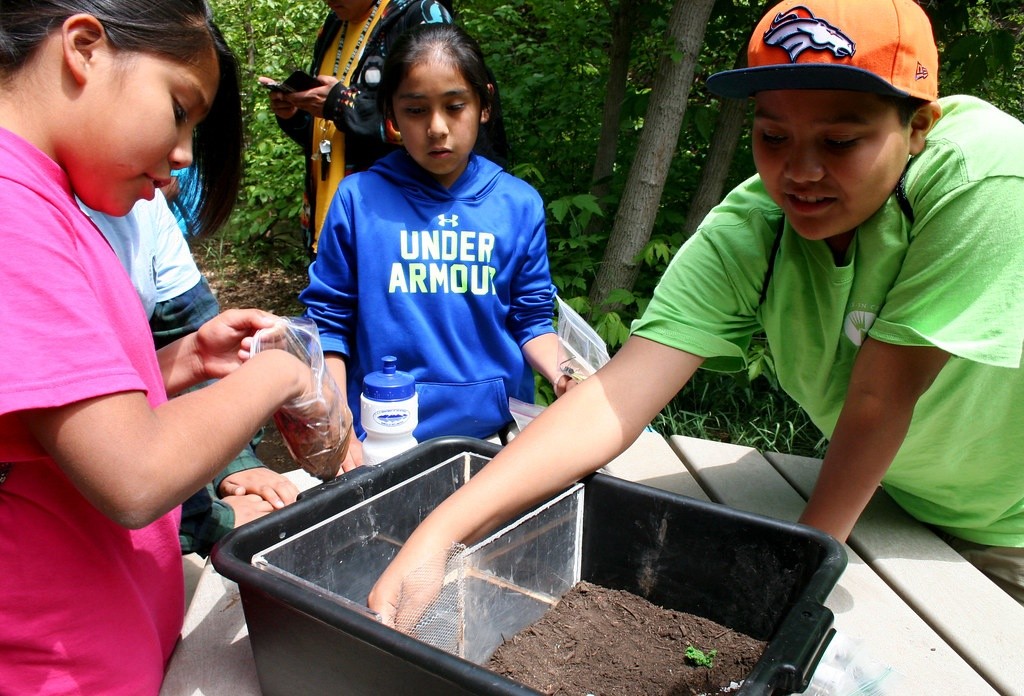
<box><xmin>210</xmin><ymin>435</ymin><xmax>848</xmax><ymax>696</ymax></box>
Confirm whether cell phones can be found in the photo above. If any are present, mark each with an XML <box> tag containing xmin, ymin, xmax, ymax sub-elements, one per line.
<box><xmin>264</xmin><ymin>70</ymin><xmax>323</xmax><ymax>95</ymax></box>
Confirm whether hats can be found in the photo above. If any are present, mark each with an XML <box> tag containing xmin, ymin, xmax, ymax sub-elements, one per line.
<box><xmin>707</xmin><ymin>0</ymin><xmax>938</xmax><ymax>102</ymax></box>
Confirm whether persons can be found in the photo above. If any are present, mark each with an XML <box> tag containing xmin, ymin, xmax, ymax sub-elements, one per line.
<box><xmin>367</xmin><ymin>0</ymin><xmax>1024</xmax><ymax>639</ymax></box>
<box><xmin>259</xmin><ymin>0</ymin><xmax>593</xmax><ymax>476</ymax></box>
<box><xmin>0</xmin><ymin>0</ymin><xmax>345</xmax><ymax>696</ymax></box>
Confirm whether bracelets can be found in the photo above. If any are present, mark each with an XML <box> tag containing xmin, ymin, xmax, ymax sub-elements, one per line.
<box><xmin>554</xmin><ymin>374</ymin><xmax>562</xmax><ymax>384</ymax></box>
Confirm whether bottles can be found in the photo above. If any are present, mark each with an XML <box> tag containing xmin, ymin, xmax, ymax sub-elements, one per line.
<box><xmin>360</xmin><ymin>355</ymin><xmax>419</xmax><ymax>467</ymax></box>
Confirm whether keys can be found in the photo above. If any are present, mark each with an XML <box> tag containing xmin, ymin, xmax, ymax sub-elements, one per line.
<box><xmin>311</xmin><ymin>139</ymin><xmax>332</xmax><ymax>163</ymax></box>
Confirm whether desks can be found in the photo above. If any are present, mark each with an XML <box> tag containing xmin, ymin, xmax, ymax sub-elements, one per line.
<box><xmin>157</xmin><ymin>432</ymin><xmax>1024</xmax><ymax>696</ymax></box>
